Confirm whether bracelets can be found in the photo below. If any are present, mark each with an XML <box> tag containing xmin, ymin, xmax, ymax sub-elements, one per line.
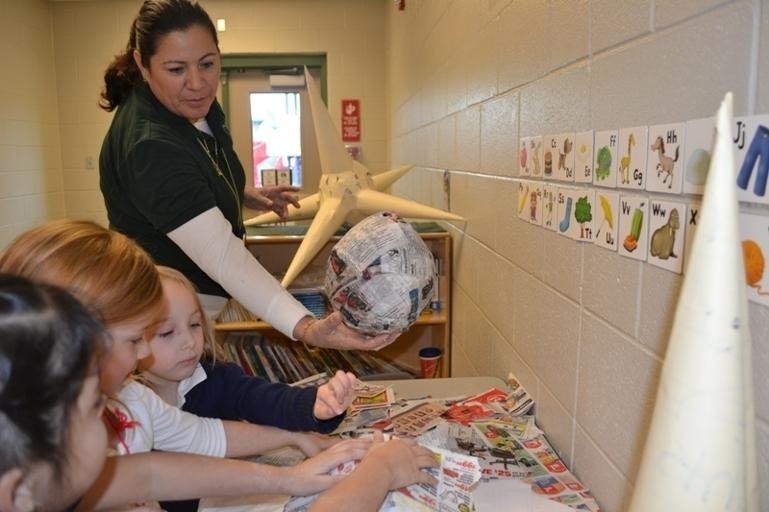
<box><xmin>302</xmin><ymin>318</ymin><xmax>319</xmax><ymax>354</ymax></box>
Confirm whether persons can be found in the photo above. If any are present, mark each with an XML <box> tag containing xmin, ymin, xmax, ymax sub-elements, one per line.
<box><xmin>98</xmin><ymin>1</ymin><xmax>403</xmax><ymax>354</ymax></box>
<box><xmin>137</xmin><ymin>267</ymin><xmax>360</xmax><ymax>512</ymax></box>
<box><xmin>0</xmin><ymin>270</ymin><xmax>440</xmax><ymax>511</ymax></box>
<box><xmin>1</xmin><ymin>215</ymin><xmax>344</xmax><ymax>512</ymax></box>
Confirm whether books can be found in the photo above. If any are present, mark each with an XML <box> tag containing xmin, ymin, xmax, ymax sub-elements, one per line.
<box><xmin>224</xmin><ymin>336</ymin><xmax>415</xmax><ymax>386</ymax></box>
<box><xmin>212</xmin><ymin>288</ymin><xmax>324</xmax><ymax>322</ymax></box>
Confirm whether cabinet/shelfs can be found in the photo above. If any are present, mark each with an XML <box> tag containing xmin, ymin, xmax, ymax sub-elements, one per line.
<box><xmin>209</xmin><ymin>217</ymin><xmax>452</xmax><ymax>378</ymax></box>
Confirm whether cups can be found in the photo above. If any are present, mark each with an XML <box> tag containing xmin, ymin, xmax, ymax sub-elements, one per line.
<box><xmin>418</xmin><ymin>346</ymin><xmax>443</xmax><ymax>379</ymax></box>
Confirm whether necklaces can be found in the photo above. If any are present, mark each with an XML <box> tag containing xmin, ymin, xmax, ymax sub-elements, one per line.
<box><xmin>201</xmin><ymin>137</ymin><xmax>223</xmax><ymax>178</ymax></box>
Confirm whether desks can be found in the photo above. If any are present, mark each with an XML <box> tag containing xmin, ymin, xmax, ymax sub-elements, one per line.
<box><xmin>194</xmin><ymin>374</ymin><xmax>600</xmax><ymax>512</ymax></box>
